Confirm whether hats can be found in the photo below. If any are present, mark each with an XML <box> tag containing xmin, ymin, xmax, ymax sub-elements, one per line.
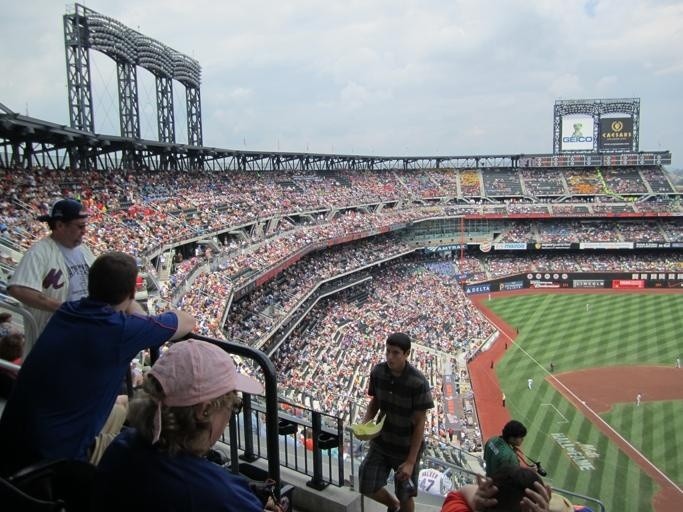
<box><xmin>146</xmin><ymin>337</ymin><xmax>264</xmax><ymax>409</ymax></box>
<box><xmin>35</xmin><ymin>198</ymin><xmax>89</xmax><ymax>223</ymax></box>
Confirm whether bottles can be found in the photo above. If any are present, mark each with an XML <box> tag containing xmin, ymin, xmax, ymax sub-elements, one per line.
<box><xmin>571</xmin><ymin>124</ymin><xmax>583</xmax><ymax>144</ymax></box>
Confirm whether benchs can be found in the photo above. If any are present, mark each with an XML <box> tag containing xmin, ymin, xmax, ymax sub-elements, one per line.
<box><xmin>1</xmin><ymin>166</ymin><xmax>683</xmax><ymax>511</ymax></box>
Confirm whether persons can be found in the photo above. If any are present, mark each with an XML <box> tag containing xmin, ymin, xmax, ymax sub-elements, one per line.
<box><xmin>0</xmin><ymin>157</ymin><xmax>682</xmax><ymax>510</ymax></box>
